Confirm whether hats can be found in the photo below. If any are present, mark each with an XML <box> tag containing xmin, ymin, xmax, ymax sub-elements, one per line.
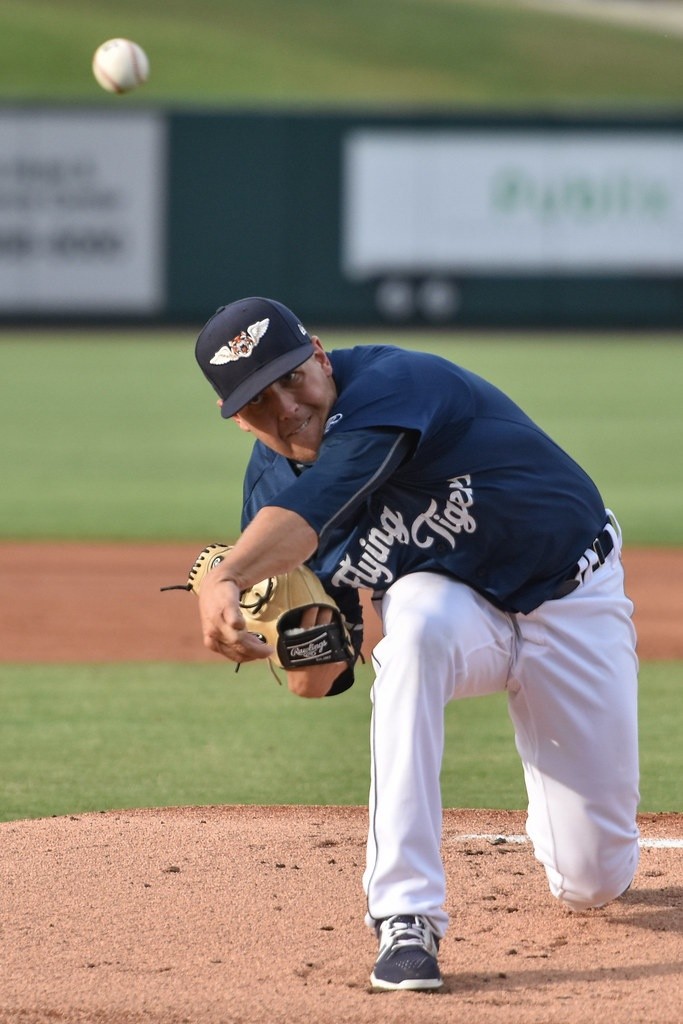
<box><xmin>195</xmin><ymin>296</ymin><xmax>314</xmax><ymax>419</ymax></box>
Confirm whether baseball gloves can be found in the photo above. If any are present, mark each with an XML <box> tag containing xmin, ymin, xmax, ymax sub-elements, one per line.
<box><xmin>186</xmin><ymin>541</ymin><xmax>358</xmax><ymax>672</ymax></box>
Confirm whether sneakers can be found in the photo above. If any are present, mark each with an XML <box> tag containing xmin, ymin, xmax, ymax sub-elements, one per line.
<box><xmin>369</xmin><ymin>914</ymin><xmax>444</xmax><ymax>992</ymax></box>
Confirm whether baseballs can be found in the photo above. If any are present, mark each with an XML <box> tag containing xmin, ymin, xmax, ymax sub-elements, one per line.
<box><xmin>91</xmin><ymin>37</ymin><xmax>150</xmax><ymax>96</ymax></box>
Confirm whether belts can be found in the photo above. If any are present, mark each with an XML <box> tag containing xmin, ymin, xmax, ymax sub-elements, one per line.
<box><xmin>546</xmin><ymin>515</ymin><xmax>614</xmax><ymax>600</ymax></box>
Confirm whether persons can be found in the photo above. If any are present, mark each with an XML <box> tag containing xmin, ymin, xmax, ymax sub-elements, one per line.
<box><xmin>194</xmin><ymin>298</ymin><xmax>640</xmax><ymax>992</ymax></box>
